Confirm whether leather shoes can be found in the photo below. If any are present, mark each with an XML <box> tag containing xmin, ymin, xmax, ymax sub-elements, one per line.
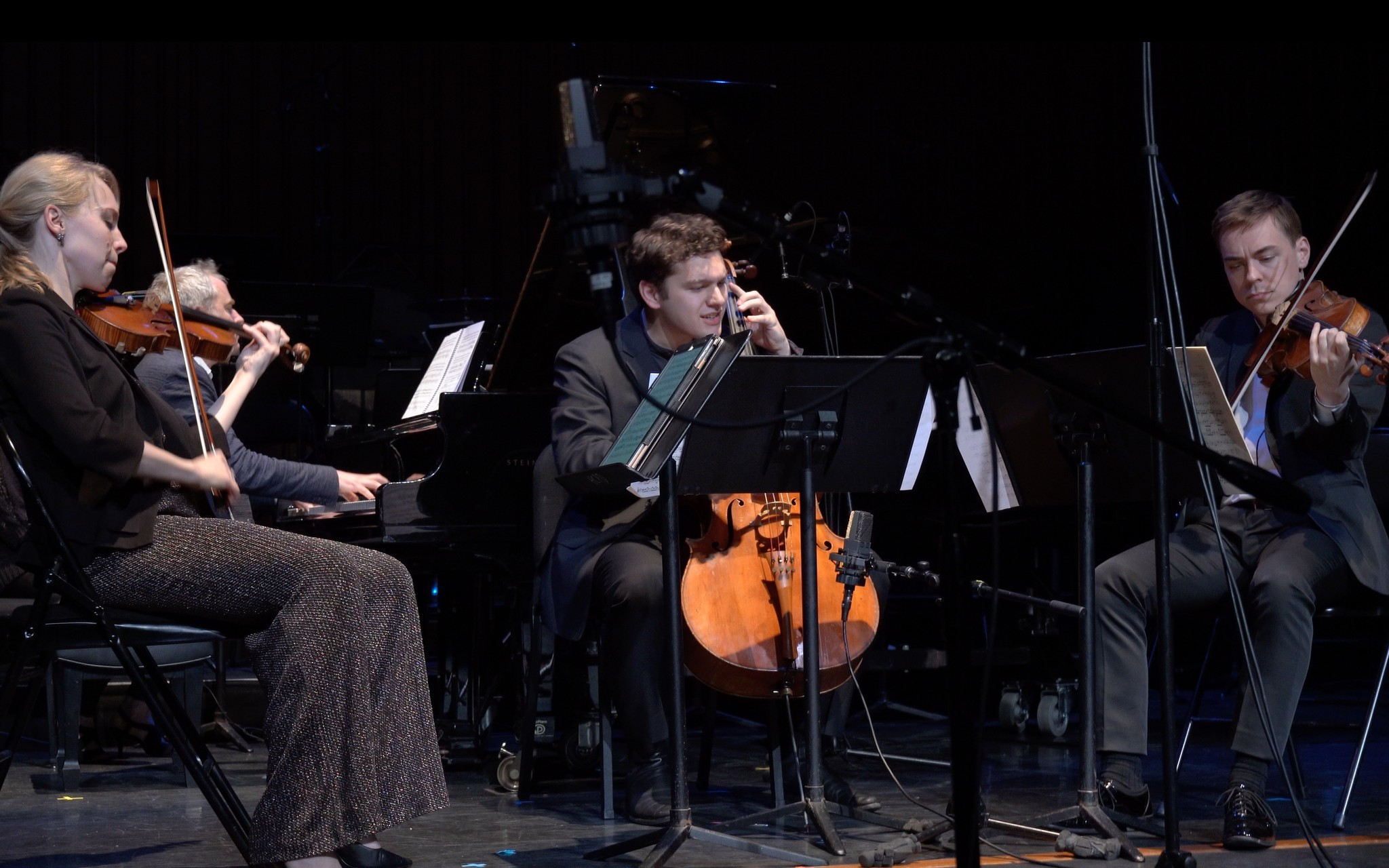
<box><xmin>1214</xmin><ymin>783</ymin><xmax>1277</xmax><ymax>847</ymax></box>
<box><xmin>797</xmin><ymin>776</ymin><xmax>881</xmax><ymax>809</ymax></box>
<box><xmin>333</xmin><ymin>842</ymin><xmax>413</xmax><ymax>868</ymax></box>
<box><xmin>596</xmin><ymin>786</ymin><xmax>673</xmax><ymax>825</ymax></box>
<box><xmin>1048</xmin><ymin>778</ymin><xmax>1154</xmax><ymax>834</ymax></box>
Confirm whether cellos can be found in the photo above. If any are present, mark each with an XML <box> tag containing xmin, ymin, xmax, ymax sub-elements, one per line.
<box><xmin>681</xmin><ymin>257</ymin><xmax>882</xmax><ymax>830</ymax></box>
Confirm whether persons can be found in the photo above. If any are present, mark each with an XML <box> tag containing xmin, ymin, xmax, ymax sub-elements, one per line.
<box><xmin>132</xmin><ymin>256</ymin><xmax>389</xmax><ymax>524</ymax></box>
<box><xmin>533</xmin><ymin>213</ymin><xmax>881</xmax><ymax>825</ymax></box>
<box><xmin>1057</xmin><ymin>189</ymin><xmax>1388</xmax><ymax>848</ymax></box>
<box><xmin>0</xmin><ymin>148</ymin><xmax>449</xmax><ymax>868</ymax></box>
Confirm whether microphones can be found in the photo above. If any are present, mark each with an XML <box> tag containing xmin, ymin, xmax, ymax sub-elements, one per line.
<box><xmin>778</xmin><ymin>242</ymin><xmax>790</xmax><ymax>279</ymax></box>
<box><xmin>838</xmin><ymin>510</ymin><xmax>874</xmax><ymax>585</ymax></box>
<box><xmin>762</xmin><ymin>204</ymin><xmax>796</xmax><ymax>252</ymax></box>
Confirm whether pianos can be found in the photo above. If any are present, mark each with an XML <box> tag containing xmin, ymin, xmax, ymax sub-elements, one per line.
<box><xmin>277</xmin><ymin>213</ymin><xmax>604</xmax><ymax>795</ymax></box>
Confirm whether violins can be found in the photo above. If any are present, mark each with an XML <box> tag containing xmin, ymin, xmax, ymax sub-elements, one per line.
<box><xmin>1246</xmin><ymin>279</ymin><xmax>1389</xmax><ymax>387</ymax></box>
<box><xmin>74</xmin><ymin>287</ymin><xmax>310</xmax><ymax>373</ymax></box>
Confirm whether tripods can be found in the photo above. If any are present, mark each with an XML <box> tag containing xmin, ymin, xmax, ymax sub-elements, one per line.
<box><xmin>573</xmin><ymin>337</ymin><xmax>832</xmax><ymax>867</ymax></box>
<box><xmin>988</xmin><ymin>342</ymin><xmax>1200</xmax><ymax>864</ymax></box>
<box><xmin>678</xmin><ymin>352</ymin><xmax>937</xmax><ymax>857</ymax></box>
